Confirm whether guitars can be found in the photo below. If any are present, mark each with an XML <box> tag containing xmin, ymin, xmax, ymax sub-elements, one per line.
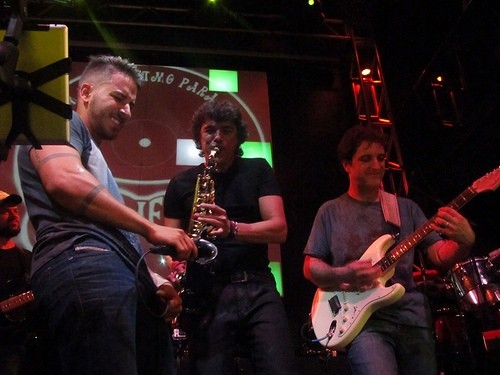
<box><xmin>311</xmin><ymin>167</ymin><xmax>500</xmax><ymax>350</ymax></box>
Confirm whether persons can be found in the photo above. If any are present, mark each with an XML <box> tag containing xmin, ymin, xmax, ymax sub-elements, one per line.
<box><xmin>0</xmin><ymin>56</ymin><xmax>476</xmax><ymax>375</ymax></box>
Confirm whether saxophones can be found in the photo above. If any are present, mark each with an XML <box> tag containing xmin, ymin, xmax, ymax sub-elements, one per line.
<box><xmin>186</xmin><ymin>146</ymin><xmax>220</xmax><ymax>264</ymax></box>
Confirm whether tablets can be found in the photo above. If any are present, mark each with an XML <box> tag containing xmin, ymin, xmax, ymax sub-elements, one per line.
<box><xmin>0</xmin><ymin>22</ymin><xmax>70</xmax><ymax>145</ymax></box>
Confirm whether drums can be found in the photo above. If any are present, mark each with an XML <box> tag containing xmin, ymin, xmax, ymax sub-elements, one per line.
<box><xmin>442</xmin><ymin>256</ymin><xmax>500</xmax><ymax>317</ymax></box>
<box><xmin>0</xmin><ymin>289</ymin><xmax>34</xmax><ymax>327</ymax></box>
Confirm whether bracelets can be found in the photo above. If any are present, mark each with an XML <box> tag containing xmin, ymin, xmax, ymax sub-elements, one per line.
<box><xmin>229</xmin><ymin>221</ymin><xmax>237</xmax><ymax>239</ymax></box>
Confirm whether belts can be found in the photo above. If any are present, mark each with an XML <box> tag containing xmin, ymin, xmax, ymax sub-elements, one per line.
<box><xmin>230</xmin><ymin>270</ymin><xmax>271</xmax><ymax>284</ymax></box>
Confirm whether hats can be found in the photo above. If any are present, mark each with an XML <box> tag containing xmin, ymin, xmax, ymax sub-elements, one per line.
<box><xmin>0</xmin><ymin>190</ymin><xmax>22</xmax><ymax>206</ymax></box>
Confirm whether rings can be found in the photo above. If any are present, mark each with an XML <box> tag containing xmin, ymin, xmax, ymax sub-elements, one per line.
<box><xmin>446</xmin><ymin>222</ymin><xmax>449</xmax><ymax>227</ymax></box>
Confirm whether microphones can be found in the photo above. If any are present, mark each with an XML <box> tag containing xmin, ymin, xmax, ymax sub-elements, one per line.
<box><xmin>149</xmin><ymin>241</ymin><xmax>207</xmax><ymax>258</ymax></box>
